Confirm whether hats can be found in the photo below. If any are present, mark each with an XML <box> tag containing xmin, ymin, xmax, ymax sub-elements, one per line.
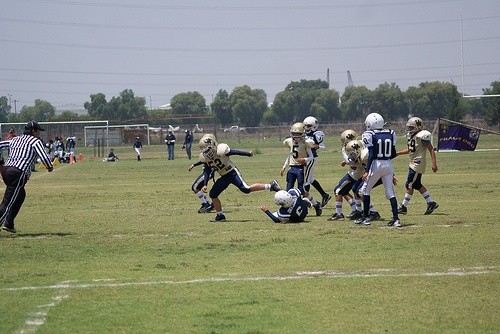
<box><xmin>25</xmin><ymin>120</ymin><xmax>45</xmax><ymax>131</ymax></box>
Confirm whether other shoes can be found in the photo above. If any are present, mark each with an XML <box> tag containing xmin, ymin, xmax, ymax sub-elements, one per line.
<box><xmin>3</xmin><ymin>223</ymin><xmax>16</xmax><ymax>233</ymax></box>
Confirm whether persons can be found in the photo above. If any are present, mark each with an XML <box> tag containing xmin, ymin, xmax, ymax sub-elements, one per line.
<box><xmin>133</xmin><ymin>136</ymin><xmax>143</xmax><ymax>161</ymax></box>
<box><xmin>181</xmin><ymin>129</ymin><xmax>193</xmax><ymax>159</ymax></box>
<box><xmin>260</xmin><ymin>113</ymin><xmax>401</xmax><ymax>227</ymax></box>
<box><xmin>164</xmin><ymin>130</ymin><xmax>176</xmax><ymax>160</ymax></box>
<box><xmin>188</xmin><ymin>134</ymin><xmax>218</xmax><ymax>214</ymax></box>
<box><xmin>4</xmin><ymin>127</ymin><xmax>77</xmax><ymax>164</ymax></box>
<box><xmin>198</xmin><ymin>137</ymin><xmax>282</xmax><ymax>222</ymax></box>
<box><xmin>107</xmin><ymin>148</ymin><xmax>119</xmax><ymax>161</ymax></box>
<box><xmin>390</xmin><ymin>117</ymin><xmax>439</xmax><ymax>215</ymax></box>
<box><xmin>0</xmin><ymin>120</ymin><xmax>53</xmax><ymax>233</ymax></box>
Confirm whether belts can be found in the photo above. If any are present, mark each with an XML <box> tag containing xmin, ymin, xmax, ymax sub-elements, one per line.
<box><xmin>377</xmin><ymin>157</ymin><xmax>389</xmax><ymax>160</ymax></box>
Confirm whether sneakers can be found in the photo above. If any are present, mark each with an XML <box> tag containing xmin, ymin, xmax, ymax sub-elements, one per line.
<box><xmin>313</xmin><ymin>201</ymin><xmax>322</xmax><ymax>216</ymax></box>
<box><xmin>303</xmin><ymin>198</ymin><xmax>312</xmax><ymax>208</ymax></box>
<box><xmin>350</xmin><ymin>209</ymin><xmax>364</xmax><ymax>220</ymax></box>
<box><xmin>346</xmin><ymin>209</ymin><xmax>358</xmax><ymax>218</ymax></box>
<box><xmin>388</xmin><ymin>219</ymin><xmax>401</xmax><ymax>227</ymax></box>
<box><xmin>364</xmin><ymin>210</ymin><xmax>381</xmax><ymax>224</ymax></box>
<box><xmin>424</xmin><ymin>201</ymin><xmax>439</xmax><ymax>215</ymax></box>
<box><xmin>354</xmin><ymin>212</ymin><xmax>370</xmax><ymax>224</ymax></box>
<box><xmin>327</xmin><ymin>213</ymin><xmax>345</xmax><ymax>221</ymax></box>
<box><xmin>269</xmin><ymin>180</ymin><xmax>281</xmax><ymax>192</ymax></box>
<box><xmin>391</xmin><ymin>203</ymin><xmax>407</xmax><ymax>214</ymax></box>
<box><xmin>198</xmin><ymin>201</ymin><xmax>213</xmax><ymax>213</ymax></box>
<box><xmin>321</xmin><ymin>194</ymin><xmax>332</xmax><ymax>207</ymax></box>
<box><xmin>209</xmin><ymin>214</ymin><xmax>226</xmax><ymax>222</ymax></box>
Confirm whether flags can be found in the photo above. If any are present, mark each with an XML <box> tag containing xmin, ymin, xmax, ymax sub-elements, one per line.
<box><xmin>438</xmin><ymin>118</ymin><xmax>481</xmax><ymax>152</ymax></box>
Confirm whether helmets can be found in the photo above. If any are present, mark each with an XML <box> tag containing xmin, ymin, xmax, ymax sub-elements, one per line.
<box><xmin>203</xmin><ymin>134</ymin><xmax>216</xmax><ymax>140</ymax></box>
<box><xmin>199</xmin><ymin>137</ymin><xmax>218</xmax><ymax>160</ymax></box>
<box><xmin>302</xmin><ymin>116</ymin><xmax>319</xmax><ymax>134</ymax></box>
<box><xmin>340</xmin><ymin>129</ymin><xmax>358</xmax><ymax>147</ymax></box>
<box><xmin>364</xmin><ymin>112</ymin><xmax>384</xmax><ymax>131</ymax></box>
<box><xmin>344</xmin><ymin>140</ymin><xmax>364</xmax><ymax>163</ymax></box>
<box><xmin>274</xmin><ymin>190</ymin><xmax>293</xmax><ymax>208</ymax></box>
<box><xmin>405</xmin><ymin>116</ymin><xmax>423</xmax><ymax>137</ymax></box>
<box><xmin>289</xmin><ymin>122</ymin><xmax>306</xmax><ymax>142</ymax></box>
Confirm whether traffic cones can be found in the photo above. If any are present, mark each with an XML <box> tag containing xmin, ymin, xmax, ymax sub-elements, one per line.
<box><xmin>78</xmin><ymin>153</ymin><xmax>83</xmax><ymax>163</ymax></box>
<box><xmin>69</xmin><ymin>155</ymin><xmax>74</xmax><ymax>165</ymax></box>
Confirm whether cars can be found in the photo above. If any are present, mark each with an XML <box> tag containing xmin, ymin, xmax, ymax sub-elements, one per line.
<box><xmin>223</xmin><ymin>125</ymin><xmax>246</xmax><ymax>133</ymax></box>
